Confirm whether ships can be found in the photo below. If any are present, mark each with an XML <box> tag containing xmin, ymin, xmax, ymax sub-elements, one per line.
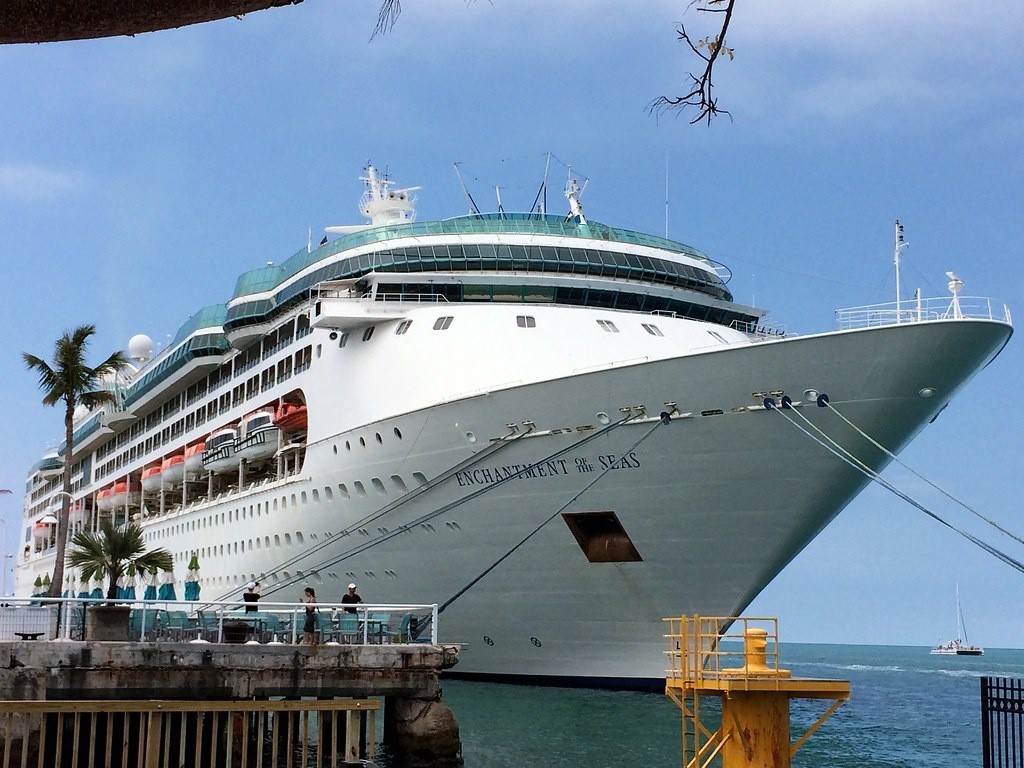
<box><xmin>7</xmin><ymin>154</ymin><xmax>1015</xmax><ymax>692</ymax></box>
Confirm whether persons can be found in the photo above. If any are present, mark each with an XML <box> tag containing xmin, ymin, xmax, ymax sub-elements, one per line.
<box><xmin>299</xmin><ymin>587</ymin><xmax>316</xmax><ymax>645</ymax></box>
<box><xmin>243</xmin><ymin>582</ymin><xmax>262</xmax><ymax>615</ymax></box>
<box><xmin>342</xmin><ymin>583</ymin><xmax>363</xmax><ymax>614</ymax></box>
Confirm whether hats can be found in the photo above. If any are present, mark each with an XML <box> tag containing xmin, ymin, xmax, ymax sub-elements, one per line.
<box><xmin>348</xmin><ymin>583</ymin><xmax>356</xmax><ymax>589</ymax></box>
<box><xmin>247</xmin><ymin>581</ymin><xmax>256</xmax><ymax>589</ymax></box>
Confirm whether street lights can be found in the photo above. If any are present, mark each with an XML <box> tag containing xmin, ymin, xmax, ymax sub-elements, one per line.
<box><xmin>38</xmin><ymin>490</ymin><xmax>77</xmax><ymax>642</ymax></box>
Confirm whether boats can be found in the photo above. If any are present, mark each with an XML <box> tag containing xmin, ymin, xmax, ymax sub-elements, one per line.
<box><xmin>272</xmin><ymin>403</ymin><xmax>308</xmax><ymax>433</ymax></box>
<box><xmin>95</xmin><ymin>490</ymin><xmax>112</xmax><ymax>513</ymax></box>
<box><xmin>159</xmin><ymin>454</ymin><xmax>190</xmax><ymax>487</ymax></box>
<box><xmin>232</xmin><ymin>408</ymin><xmax>281</xmax><ymax>461</ymax></box>
<box><xmin>201</xmin><ymin>423</ymin><xmax>245</xmax><ymax>475</ymax></box>
<box><xmin>140</xmin><ymin>464</ymin><xmax>161</xmax><ymax>494</ymax></box>
<box><xmin>183</xmin><ymin>442</ymin><xmax>209</xmax><ymax>473</ymax></box>
<box><xmin>109</xmin><ymin>480</ymin><xmax>131</xmax><ymax>507</ymax></box>
<box><xmin>67</xmin><ymin>505</ymin><xmax>79</xmax><ymax>525</ymax></box>
<box><xmin>30</xmin><ymin>523</ymin><xmax>53</xmax><ymax>541</ymax></box>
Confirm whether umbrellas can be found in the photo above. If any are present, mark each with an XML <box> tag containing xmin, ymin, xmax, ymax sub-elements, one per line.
<box><xmin>158</xmin><ymin>571</ymin><xmax>177</xmax><ymax>610</ymax></box>
<box><xmin>64</xmin><ymin>575</ymin><xmax>76</xmax><ymax>598</ymax></box>
<box><xmin>185</xmin><ymin>552</ymin><xmax>200</xmax><ymax>612</ymax></box>
<box><xmin>31</xmin><ymin>577</ymin><xmax>42</xmax><ymax>606</ymax></box>
<box><xmin>90</xmin><ymin>580</ymin><xmax>105</xmax><ymax>606</ymax></box>
<box><xmin>115</xmin><ymin>577</ymin><xmax>124</xmax><ymax>606</ymax></box>
<box><xmin>42</xmin><ymin>575</ymin><xmax>51</xmax><ymax>597</ymax></box>
<box><xmin>124</xmin><ymin>576</ymin><xmax>137</xmax><ymax>607</ymax></box>
<box><xmin>145</xmin><ymin>573</ymin><xmax>159</xmax><ymax>608</ymax></box>
<box><xmin>77</xmin><ymin>582</ymin><xmax>89</xmax><ymax>598</ymax></box>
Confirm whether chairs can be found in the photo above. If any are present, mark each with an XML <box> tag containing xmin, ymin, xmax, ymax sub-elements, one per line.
<box><xmin>54</xmin><ymin>609</ymin><xmax>414</xmax><ymax>644</ymax></box>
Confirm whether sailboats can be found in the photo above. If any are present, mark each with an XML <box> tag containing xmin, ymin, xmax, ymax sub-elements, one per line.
<box><xmin>927</xmin><ymin>579</ymin><xmax>986</xmax><ymax>657</ymax></box>
